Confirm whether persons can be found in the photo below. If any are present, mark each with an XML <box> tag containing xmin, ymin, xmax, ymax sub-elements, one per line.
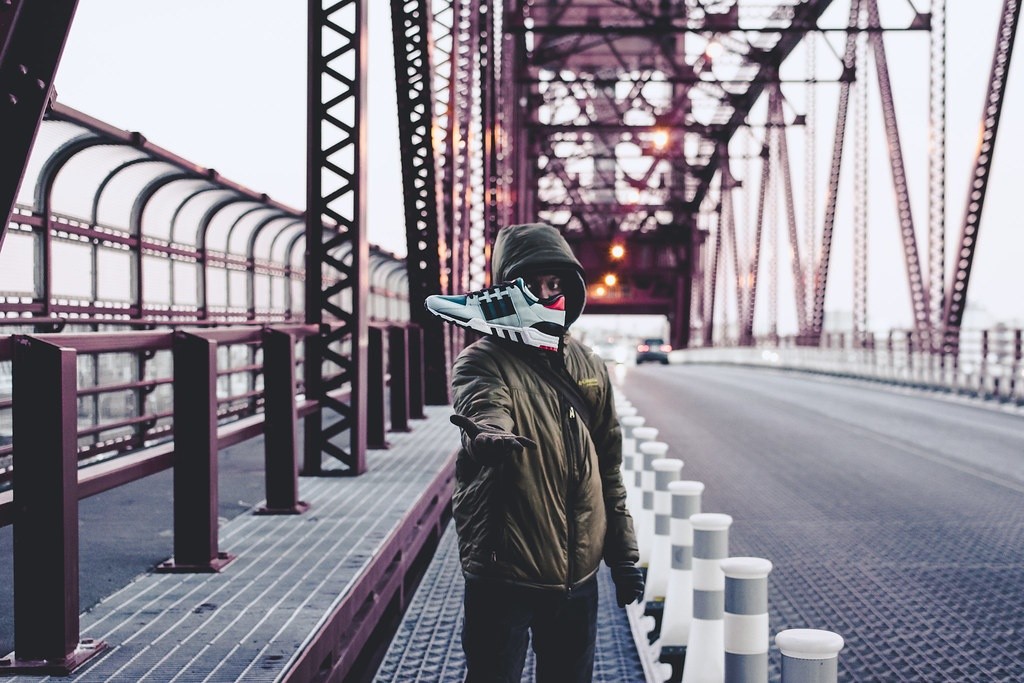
<box><xmin>451</xmin><ymin>222</ymin><xmax>649</xmax><ymax>683</ymax></box>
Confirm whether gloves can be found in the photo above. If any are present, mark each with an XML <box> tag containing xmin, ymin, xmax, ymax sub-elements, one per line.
<box><xmin>612</xmin><ymin>568</ymin><xmax>644</xmax><ymax>608</ymax></box>
<box><xmin>450</xmin><ymin>414</ymin><xmax>537</xmax><ymax>463</ymax></box>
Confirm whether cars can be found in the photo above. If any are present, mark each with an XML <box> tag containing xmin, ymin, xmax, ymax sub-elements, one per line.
<box><xmin>635</xmin><ymin>338</ymin><xmax>670</xmax><ymax>365</ymax></box>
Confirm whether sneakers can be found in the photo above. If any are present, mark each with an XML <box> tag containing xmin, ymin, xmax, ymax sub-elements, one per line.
<box><xmin>424</xmin><ymin>277</ymin><xmax>565</xmax><ymax>353</ymax></box>
